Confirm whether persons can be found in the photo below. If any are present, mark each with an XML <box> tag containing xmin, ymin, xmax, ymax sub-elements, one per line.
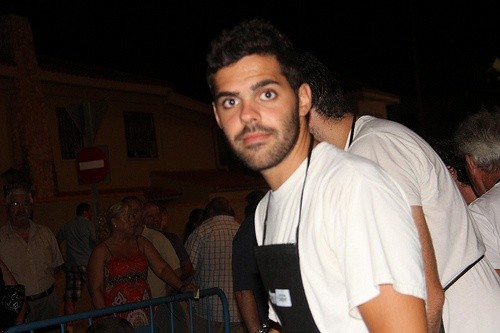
<box><xmin>0</xmin><ymin>187</ymin><xmax>282</xmax><ymax>333</ymax></box>
<box><xmin>295</xmin><ymin>52</ymin><xmax>500</xmax><ymax>333</ymax></box>
<box><xmin>207</xmin><ymin>19</ymin><xmax>428</xmax><ymax>333</ymax></box>
<box><xmin>433</xmin><ymin>104</ymin><xmax>500</xmax><ymax>273</ymax></box>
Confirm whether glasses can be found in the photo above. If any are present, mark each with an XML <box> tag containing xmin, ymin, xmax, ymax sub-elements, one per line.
<box><xmin>9</xmin><ymin>200</ymin><xmax>31</xmax><ymax>209</ymax></box>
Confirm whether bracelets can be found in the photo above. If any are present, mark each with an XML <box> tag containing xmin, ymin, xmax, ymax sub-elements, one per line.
<box><xmin>177</xmin><ymin>283</ymin><xmax>184</xmax><ymax>294</ymax></box>
<box><xmin>255</xmin><ymin>329</ymin><xmax>264</xmax><ymax>333</ymax></box>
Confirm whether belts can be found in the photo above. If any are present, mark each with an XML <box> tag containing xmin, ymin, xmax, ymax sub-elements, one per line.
<box><xmin>26</xmin><ymin>286</ymin><xmax>54</xmax><ymax>301</ymax></box>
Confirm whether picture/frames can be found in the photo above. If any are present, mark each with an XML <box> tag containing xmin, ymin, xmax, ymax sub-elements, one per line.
<box><xmin>120</xmin><ymin>109</ymin><xmax>161</xmax><ymax>161</ymax></box>
<box><xmin>213</xmin><ymin>127</ymin><xmax>230</xmax><ymax>169</ymax></box>
